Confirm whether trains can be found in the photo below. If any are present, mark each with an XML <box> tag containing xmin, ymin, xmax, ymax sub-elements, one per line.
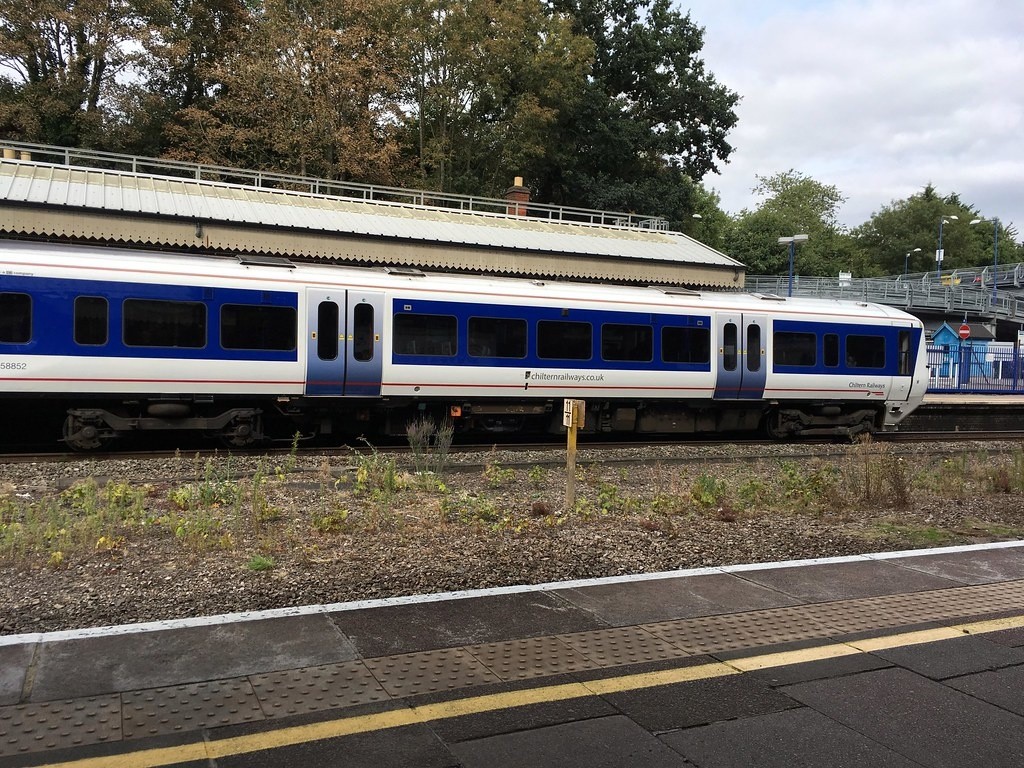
<box><xmin>0</xmin><ymin>244</ymin><xmax>928</xmax><ymax>449</ymax></box>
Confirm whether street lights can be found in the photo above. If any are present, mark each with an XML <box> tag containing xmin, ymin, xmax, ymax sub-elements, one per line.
<box><xmin>970</xmin><ymin>219</ymin><xmax>998</xmax><ymax>306</ymax></box>
<box><xmin>777</xmin><ymin>234</ymin><xmax>809</xmax><ymax>298</ymax></box>
<box><xmin>906</xmin><ymin>248</ymin><xmax>922</xmax><ymax>278</ymax></box>
<box><xmin>937</xmin><ymin>215</ymin><xmax>959</xmax><ymax>282</ymax></box>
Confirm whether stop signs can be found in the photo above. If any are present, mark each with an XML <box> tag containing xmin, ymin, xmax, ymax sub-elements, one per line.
<box><xmin>959</xmin><ymin>325</ymin><xmax>970</xmax><ymax>339</ymax></box>
